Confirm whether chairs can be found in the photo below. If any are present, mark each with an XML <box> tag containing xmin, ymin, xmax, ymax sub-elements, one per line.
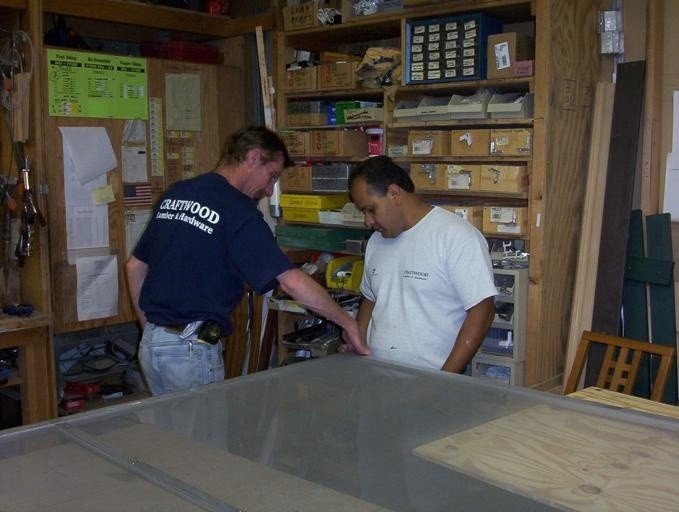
<box><xmin>563</xmin><ymin>329</ymin><xmax>674</xmax><ymax>401</ymax></box>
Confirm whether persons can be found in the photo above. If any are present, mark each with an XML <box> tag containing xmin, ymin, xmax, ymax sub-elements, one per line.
<box><xmin>336</xmin><ymin>155</ymin><xmax>497</xmax><ymax>377</ymax></box>
<box><xmin>124</xmin><ymin>122</ymin><xmax>372</xmax><ymax>398</ymax></box>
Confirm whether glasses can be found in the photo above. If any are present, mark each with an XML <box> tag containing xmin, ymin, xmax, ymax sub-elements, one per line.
<box><xmin>268</xmin><ymin>167</ymin><xmax>280</xmax><ymax>186</ymax></box>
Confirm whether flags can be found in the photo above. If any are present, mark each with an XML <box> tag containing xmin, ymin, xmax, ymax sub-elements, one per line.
<box><xmin>123</xmin><ymin>184</ymin><xmax>153</xmax><ymax>207</ymax></box>
